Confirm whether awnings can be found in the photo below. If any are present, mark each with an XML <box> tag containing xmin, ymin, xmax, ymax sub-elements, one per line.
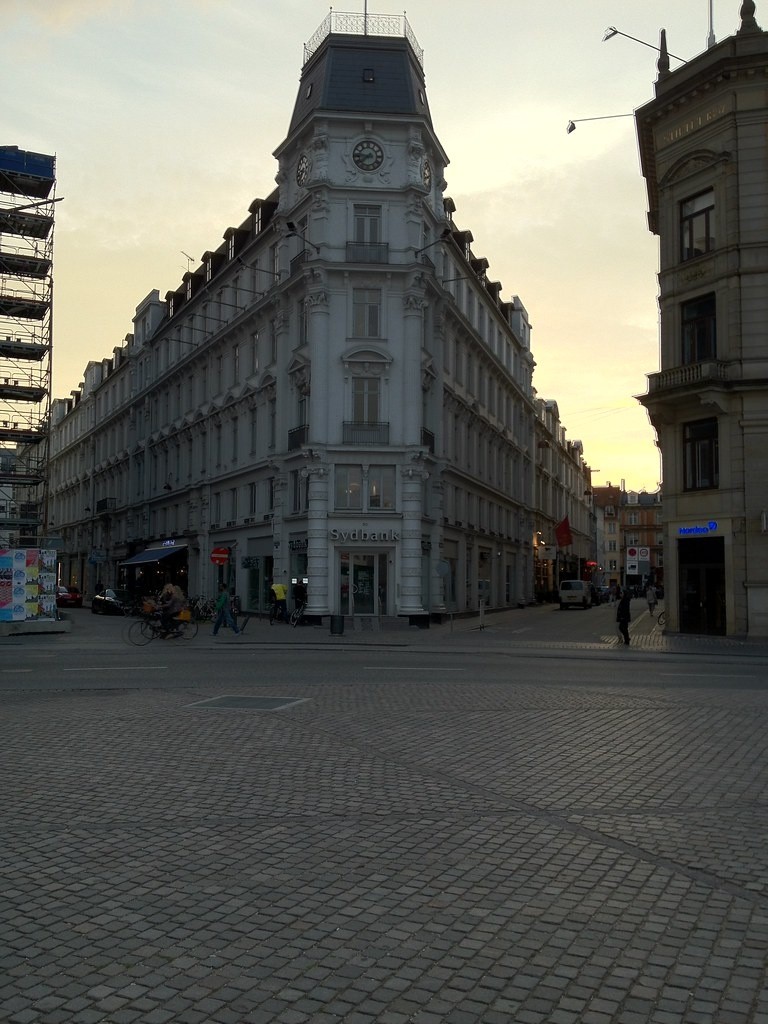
<box><xmin>118</xmin><ymin>546</ymin><xmax>185</xmax><ymax>565</ymax></box>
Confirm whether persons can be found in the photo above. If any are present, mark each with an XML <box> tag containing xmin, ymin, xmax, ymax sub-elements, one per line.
<box><xmin>271</xmin><ymin>579</ymin><xmax>290</xmax><ymax>624</ymax></box>
<box><xmin>209</xmin><ymin>583</ymin><xmax>241</xmax><ymax>637</ymax></box>
<box><xmin>157</xmin><ymin>583</ymin><xmax>187</xmax><ymax>639</ymax></box>
<box><xmin>634</xmin><ymin>584</ymin><xmax>645</xmax><ymax>599</ymax></box>
<box><xmin>608</xmin><ymin>582</ymin><xmax>616</xmax><ymax>607</ymax></box>
<box><xmin>647</xmin><ymin>586</ymin><xmax>656</xmax><ymax>616</ymax></box>
<box><xmin>617</xmin><ymin>590</ymin><xmax>631</xmax><ymax>645</ymax></box>
<box><xmin>94</xmin><ymin>580</ymin><xmax>104</xmax><ymax>595</ymax></box>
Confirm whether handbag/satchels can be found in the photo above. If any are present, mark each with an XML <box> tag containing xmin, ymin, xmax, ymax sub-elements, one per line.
<box><xmin>654</xmin><ymin>600</ymin><xmax>658</xmax><ymax>605</ymax></box>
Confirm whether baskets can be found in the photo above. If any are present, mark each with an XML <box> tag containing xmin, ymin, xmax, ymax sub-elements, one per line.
<box><xmin>143</xmin><ymin>602</ymin><xmax>155</xmax><ymax>613</ymax></box>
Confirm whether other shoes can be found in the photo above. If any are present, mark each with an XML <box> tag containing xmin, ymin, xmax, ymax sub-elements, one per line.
<box><xmin>624</xmin><ymin>638</ymin><xmax>630</xmax><ymax>645</ymax></box>
<box><xmin>235</xmin><ymin>633</ymin><xmax>240</xmax><ymax>636</ymax></box>
<box><xmin>166</xmin><ymin>634</ymin><xmax>174</xmax><ymax>639</ymax></box>
<box><xmin>210</xmin><ymin>633</ymin><xmax>216</xmax><ymax>636</ymax></box>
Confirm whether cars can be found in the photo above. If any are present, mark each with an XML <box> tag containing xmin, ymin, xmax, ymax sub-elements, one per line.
<box><xmin>91</xmin><ymin>588</ymin><xmax>137</xmax><ymax>616</ymax></box>
<box><xmin>591</xmin><ymin>583</ymin><xmax>612</xmax><ymax>606</ymax></box>
<box><xmin>54</xmin><ymin>586</ymin><xmax>84</xmax><ymax>608</ymax></box>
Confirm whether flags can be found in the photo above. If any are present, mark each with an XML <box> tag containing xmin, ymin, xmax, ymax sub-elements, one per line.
<box><xmin>555</xmin><ymin>514</ymin><xmax>572</xmax><ymax>548</ymax></box>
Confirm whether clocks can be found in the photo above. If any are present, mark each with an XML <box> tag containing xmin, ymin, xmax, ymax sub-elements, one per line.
<box><xmin>352</xmin><ymin>139</ymin><xmax>385</xmax><ymax>171</ymax></box>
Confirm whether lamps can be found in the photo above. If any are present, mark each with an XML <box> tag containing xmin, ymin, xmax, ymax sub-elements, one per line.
<box><xmin>567</xmin><ymin>111</ymin><xmax>636</xmax><ymax>135</ymax></box>
<box><xmin>601</xmin><ymin>26</ymin><xmax>688</xmax><ymax>65</ymax></box>
<box><xmin>442</xmin><ymin>269</ymin><xmax>485</xmax><ymax>287</ymax></box>
<box><xmin>414</xmin><ymin>228</ymin><xmax>451</xmax><ymax>259</ymax></box>
<box><xmin>162</xmin><ymin>222</ymin><xmax>321</xmax><ymax>345</ymax></box>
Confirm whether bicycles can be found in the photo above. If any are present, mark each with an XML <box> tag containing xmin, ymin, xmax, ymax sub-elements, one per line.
<box><xmin>128</xmin><ymin>588</ymin><xmax>240</xmax><ymax>646</ymax></box>
<box><xmin>268</xmin><ymin>597</ymin><xmax>287</xmax><ymax>626</ymax></box>
<box><xmin>290</xmin><ymin>597</ymin><xmax>307</xmax><ymax>628</ymax></box>
<box><xmin>657</xmin><ymin>611</ymin><xmax>666</xmax><ymax>625</ymax></box>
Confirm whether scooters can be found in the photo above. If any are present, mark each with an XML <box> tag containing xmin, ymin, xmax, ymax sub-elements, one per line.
<box><xmin>623</xmin><ymin>582</ymin><xmax>664</xmax><ymax>599</ymax></box>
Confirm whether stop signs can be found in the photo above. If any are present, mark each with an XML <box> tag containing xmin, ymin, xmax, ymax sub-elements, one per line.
<box><xmin>210</xmin><ymin>546</ymin><xmax>230</xmax><ymax>565</ymax></box>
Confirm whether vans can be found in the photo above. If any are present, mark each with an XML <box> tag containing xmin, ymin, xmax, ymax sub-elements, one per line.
<box><xmin>559</xmin><ymin>579</ymin><xmax>593</xmax><ymax>609</ymax></box>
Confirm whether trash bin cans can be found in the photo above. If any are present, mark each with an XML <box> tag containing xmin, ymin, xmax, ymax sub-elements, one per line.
<box><xmin>330</xmin><ymin>615</ymin><xmax>344</xmax><ymax>634</ymax></box>
